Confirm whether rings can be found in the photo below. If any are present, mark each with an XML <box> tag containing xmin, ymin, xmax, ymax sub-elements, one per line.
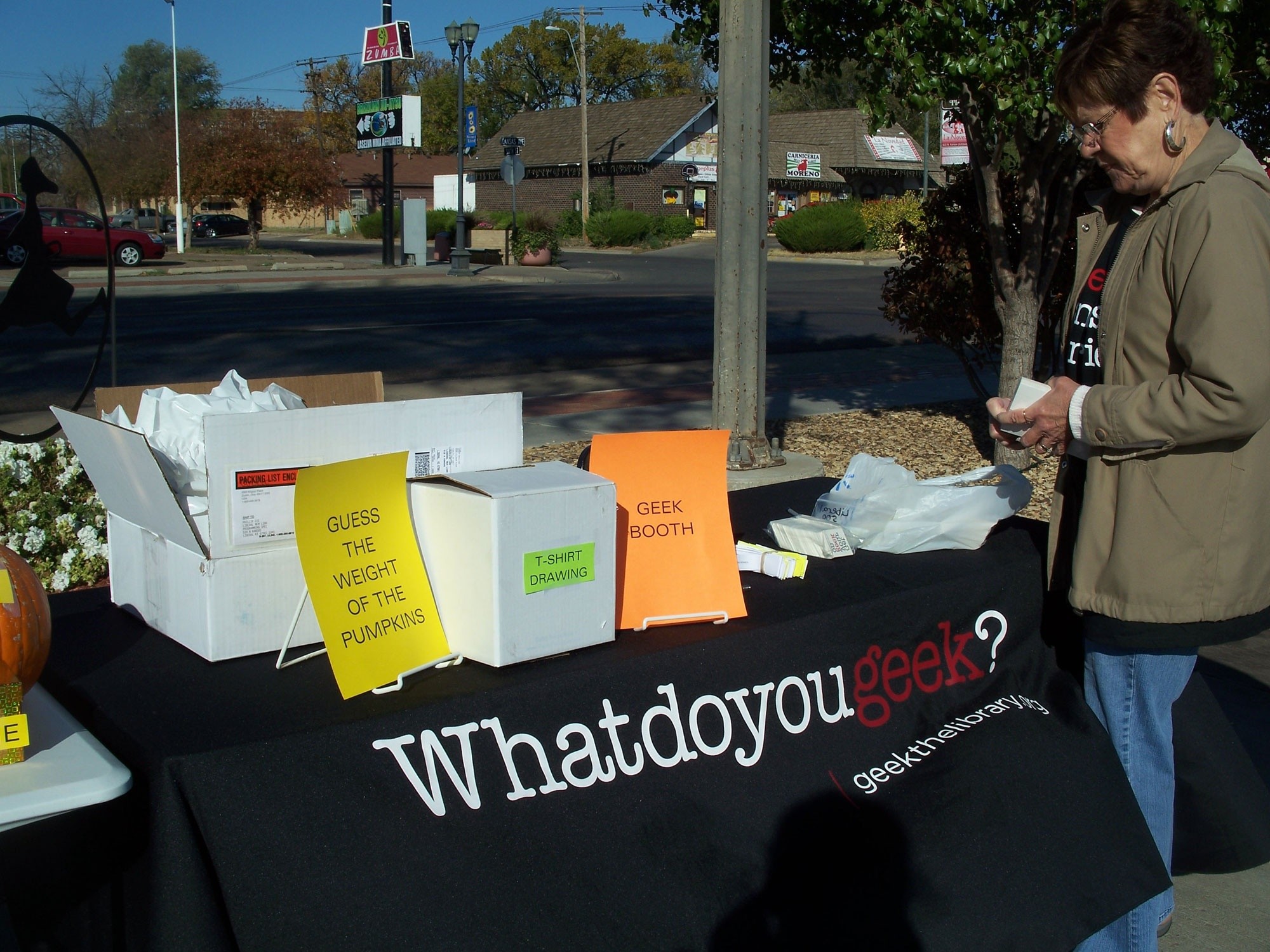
<box><xmin>1023</xmin><ymin>408</ymin><xmax>1029</xmax><ymax>423</ymax></box>
<box><xmin>1039</xmin><ymin>441</ymin><xmax>1050</xmax><ymax>452</ymax></box>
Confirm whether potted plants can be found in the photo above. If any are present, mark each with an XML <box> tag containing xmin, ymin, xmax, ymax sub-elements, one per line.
<box><xmin>509</xmin><ymin>226</ymin><xmax>561</xmax><ymax>265</ymax></box>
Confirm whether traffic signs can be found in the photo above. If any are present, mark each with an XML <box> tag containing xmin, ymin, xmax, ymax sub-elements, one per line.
<box><xmin>500</xmin><ymin>136</ymin><xmax>526</xmax><ymax>147</ymax></box>
<box><xmin>504</xmin><ymin>146</ymin><xmax>519</xmax><ymax>156</ymax></box>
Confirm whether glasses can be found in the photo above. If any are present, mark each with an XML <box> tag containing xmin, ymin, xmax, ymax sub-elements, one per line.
<box><xmin>1072</xmin><ymin>103</ymin><xmax>1122</xmax><ymax>146</ymax></box>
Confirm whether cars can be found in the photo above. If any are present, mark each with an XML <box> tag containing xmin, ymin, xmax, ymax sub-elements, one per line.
<box><xmin>166</xmin><ymin>214</ymin><xmax>215</xmax><ymax>234</ymax></box>
<box><xmin>1</xmin><ymin>207</ymin><xmax>170</xmax><ymax>267</ymax></box>
<box><xmin>191</xmin><ymin>214</ymin><xmax>262</xmax><ymax>238</ymax></box>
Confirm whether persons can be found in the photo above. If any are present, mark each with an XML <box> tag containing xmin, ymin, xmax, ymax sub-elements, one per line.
<box><xmin>984</xmin><ymin>1</ymin><xmax>1270</xmax><ymax>947</ymax></box>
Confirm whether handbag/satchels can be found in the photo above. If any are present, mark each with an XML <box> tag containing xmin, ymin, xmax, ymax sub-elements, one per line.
<box><xmin>811</xmin><ymin>452</ymin><xmax>1033</xmax><ymax>555</ymax></box>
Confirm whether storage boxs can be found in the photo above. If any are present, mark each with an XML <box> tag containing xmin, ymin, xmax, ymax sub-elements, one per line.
<box><xmin>404</xmin><ymin>460</ymin><xmax>616</xmax><ymax>670</ymax></box>
<box><xmin>48</xmin><ymin>370</ymin><xmax>523</xmax><ymax>665</ymax></box>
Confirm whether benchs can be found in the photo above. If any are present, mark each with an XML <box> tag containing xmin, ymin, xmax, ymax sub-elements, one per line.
<box><xmin>450</xmin><ymin>245</ymin><xmax>503</xmax><ymax>265</ymax></box>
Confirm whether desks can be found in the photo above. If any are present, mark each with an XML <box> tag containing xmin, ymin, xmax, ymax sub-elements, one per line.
<box><xmin>0</xmin><ymin>471</ymin><xmax>1174</xmax><ymax>951</ymax></box>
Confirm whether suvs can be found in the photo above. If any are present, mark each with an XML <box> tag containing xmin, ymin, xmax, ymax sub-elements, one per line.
<box><xmin>106</xmin><ymin>206</ymin><xmax>177</xmax><ymax>232</ymax></box>
<box><xmin>0</xmin><ymin>192</ymin><xmax>88</xmax><ymax>228</ymax></box>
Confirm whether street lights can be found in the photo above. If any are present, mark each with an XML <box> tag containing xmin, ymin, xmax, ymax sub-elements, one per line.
<box><xmin>442</xmin><ymin>14</ymin><xmax>481</xmax><ymax>278</ymax></box>
<box><xmin>545</xmin><ymin>23</ymin><xmax>589</xmax><ymax>244</ymax></box>
